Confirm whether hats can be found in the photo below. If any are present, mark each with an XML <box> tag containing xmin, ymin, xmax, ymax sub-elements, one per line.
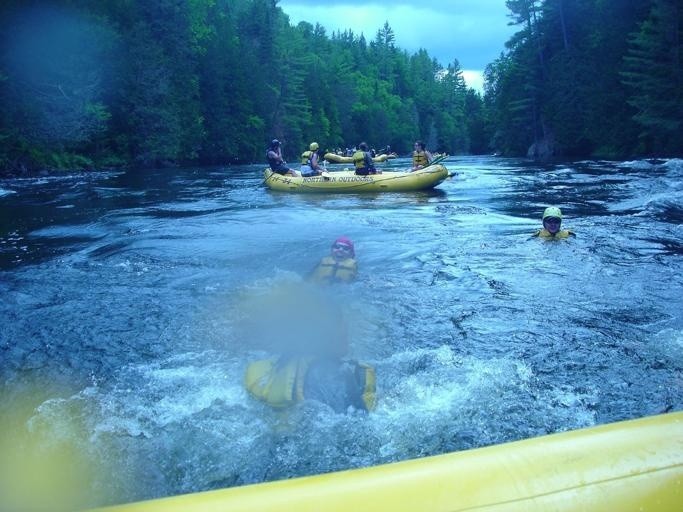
<box><xmin>336</xmin><ymin>238</ymin><xmax>352</xmax><ymax>247</ymax></box>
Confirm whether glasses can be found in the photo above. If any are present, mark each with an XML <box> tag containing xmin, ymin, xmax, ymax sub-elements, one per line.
<box><xmin>332</xmin><ymin>244</ymin><xmax>350</xmax><ymax>251</ymax></box>
<box><xmin>544</xmin><ymin>217</ymin><xmax>561</xmax><ymax>224</ymax></box>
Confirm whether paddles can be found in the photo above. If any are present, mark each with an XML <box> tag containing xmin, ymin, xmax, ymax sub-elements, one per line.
<box><xmin>319</xmin><ymin>170</ymin><xmax>330</xmax><ymax>178</ymax></box>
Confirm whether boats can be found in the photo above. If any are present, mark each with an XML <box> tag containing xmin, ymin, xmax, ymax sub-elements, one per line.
<box><xmin>264</xmin><ymin>164</ymin><xmax>448</xmax><ymax>194</ymax></box>
<box><xmin>323</xmin><ymin>152</ymin><xmax>397</xmax><ymax>163</ymax></box>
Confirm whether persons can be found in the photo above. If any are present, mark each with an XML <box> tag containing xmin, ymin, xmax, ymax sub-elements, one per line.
<box><xmin>301</xmin><ymin>142</ymin><xmax>328</xmax><ymax>177</ymax></box>
<box><xmin>408</xmin><ymin>140</ymin><xmax>434</xmax><ymax>172</ymax></box>
<box><xmin>326</xmin><ymin>144</ymin><xmax>392</xmax><ymax>157</ymax></box>
<box><xmin>303</xmin><ymin>236</ymin><xmax>358</xmax><ymax>286</ymax></box>
<box><xmin>526</xmin><ymin>204</ymin><xmax>576</xmax><ymax>242</ymax></box>
<box><xmin>353</xmin><ymin>141</ymin><xmax>377</xmax><ymax>177</ymax></box>
<box><xmin>264</xmin><ymin>138</ymin><xmax>297</xmax><ymax>177</ymax></box>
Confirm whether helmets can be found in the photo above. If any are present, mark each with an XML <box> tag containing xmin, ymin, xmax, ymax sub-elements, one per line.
<box><xmin>310</xmin><ymin>142</ymin><xmax>319</xmax><ymax>151</ymax></box>
<box><xmin>358</xmin><ymin>142</ymin><xmax>369</xmax><ymax>152</ymax></box>
<box><xmin>272</xmin><ymin>139</ymin><xmax>281</xmax><ymax>145</ymax></box>
<box><xmin>542</xmin><ymin>207</ymin><xmax>562</xmax><ymax>227</ymax></box>
